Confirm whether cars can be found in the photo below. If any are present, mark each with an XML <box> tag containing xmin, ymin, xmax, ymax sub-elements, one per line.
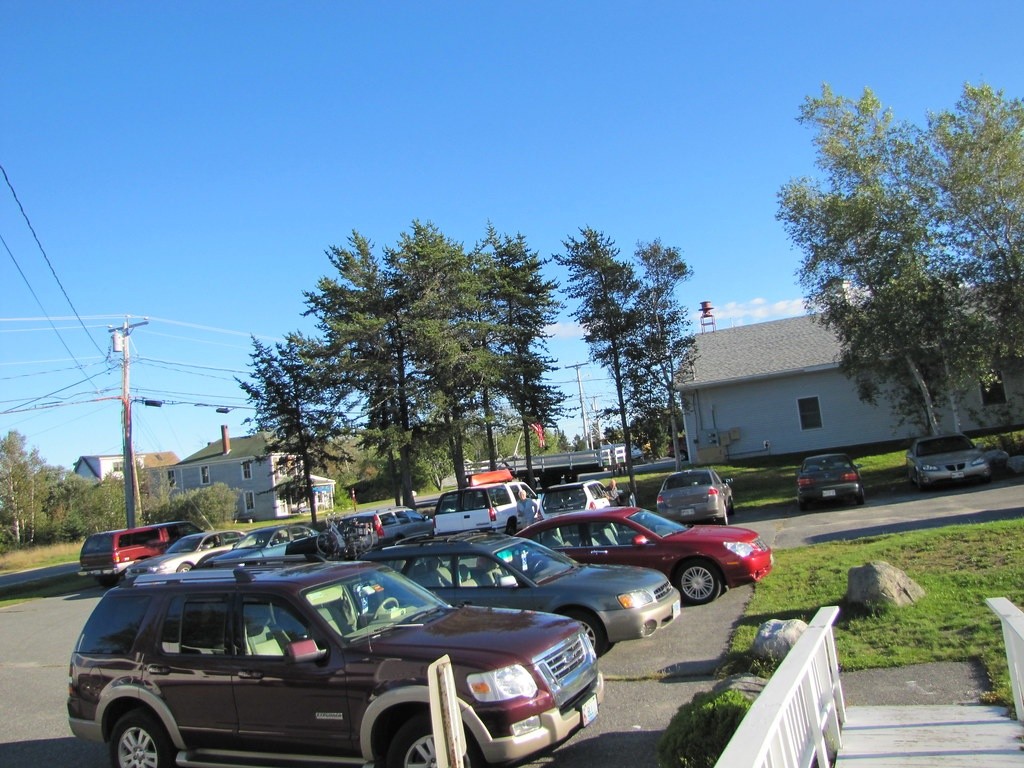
<box><xmin>356</xmin><ymin>527</ymin><xmax>681</xmax><ymax>661</ymax></box>
<box><xmin>656</xmin><ymin>469</ymin><xmax>734</xmax><ymax>526</ymax></box>
<box><xmin>905</xmin><ymin>432</ymin><xmax>991</xmax><ymax>492</ymax></box>
<box><xmin>510</xmin><ymin>506</ymin><xmax>774</xmax><ymax>605</ymax></box>
<box><xmin>124</xmin><ymin>530</ymin><xmax>256</xmax><ymax>577</ymax></box>
<box><xmin>212</xmin><ymin>525</ymin><xmax>319</xmax><ymax>567</ymax></box>
<box><xmin>794</xmin><ymin>453</ymin><xmax>866</xmax><ymax>511</ymax></box>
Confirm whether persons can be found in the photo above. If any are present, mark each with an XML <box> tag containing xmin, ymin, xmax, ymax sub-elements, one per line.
<box><xmin>517</xmin><ymin>490</ymin><xmax>537</xmax><ymax>529</ymax></box>
<box><xmin>603</xmin><ymin>480</ymin><xmax>620</xmax><ymax>506</ymax></box>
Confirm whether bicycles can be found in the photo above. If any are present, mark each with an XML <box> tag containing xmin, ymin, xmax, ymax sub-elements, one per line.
<box><xmin>315</xmin><ymin>513</ymin><xmax>374</xmax><ymax>554</ymax></box>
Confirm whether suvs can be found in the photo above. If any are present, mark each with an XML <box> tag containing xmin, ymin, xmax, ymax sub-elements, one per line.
<box><xmin>341</xmin><ymin>506</ymin><xmax>434</xmax><ymax>551</ymax></box>
<box><xmin>534</xmin><ymin>480</ymin><xmax>612</xmax><ymax>524</ymax></box>
<box><xmin>72</xmin><ymin>652</ymin><xmax>282</xmax><ymax>705</ymax></box>
<box><xmin>67</xmin><ymin>554</ymin><xmax>604</xmax><ymax>768</ymax></box>
<box><xmin>668</xmin><ymin>437</ymin><xmax>688</xmax><ymax>461</ymax></box>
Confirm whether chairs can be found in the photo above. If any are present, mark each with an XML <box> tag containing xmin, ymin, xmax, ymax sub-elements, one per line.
<box><xmin>313</xmin><ymin>607</ymin><xmax>356</xmax><ymax>638</ymax></box>
<box><xmin>460</xmin><ymin>564</ymin><xmax>479</xmax><ymax>586</ymax></box>
<box><xmin>601</xmin><ymin>526</ymin><xmax>619</xmax><ymax>545</ymax></box>
<box><xmin>243</xmin><ymin>616</ymin><xmax>290</xmax><ymax>656</ymax></box>
<box><xmin>482</xmin><ymin>557</ymin><xmax>509</xmax><ymax>586</ymax></box>
<box><xmin>580</xmin><ymin>538</ymin><xmax>602</xmax><ymax>546</ymax></box>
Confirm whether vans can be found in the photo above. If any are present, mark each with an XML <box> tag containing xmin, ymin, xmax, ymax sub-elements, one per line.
<box><xmin>76</xmin><ymin>521</ymin><xmax>209</xmax><ymax>587</ymax></box>
<box><xmin>432</xmin><ymin>469</ymin><xmax>541</xmax><ymax>538</ymax></box>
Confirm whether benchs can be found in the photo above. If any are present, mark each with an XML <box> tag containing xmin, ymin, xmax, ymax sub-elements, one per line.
<box><xmin>542</xmin><ymin>529</ymin><xmax>573</xmax><ymax>547</ymax></box>
<box><xmin>408</xmin><ymin>557</ymin><xmax>452</xmax><ymax>586</ymax></box>
